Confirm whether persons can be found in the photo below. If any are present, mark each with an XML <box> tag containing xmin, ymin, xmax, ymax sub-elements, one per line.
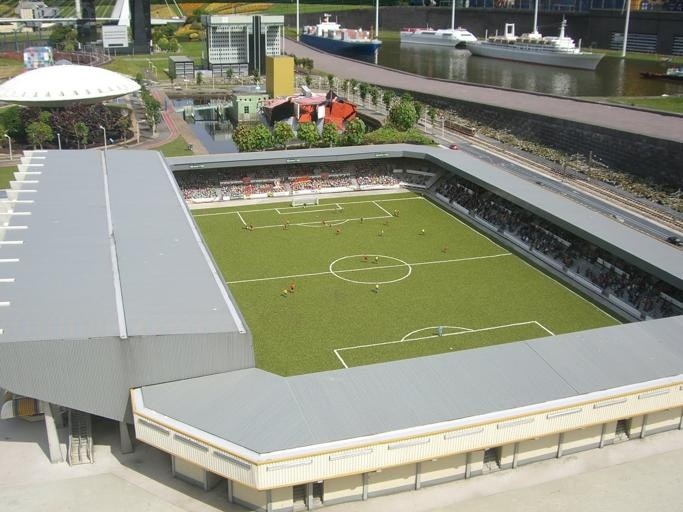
<box><xmin>436</xmin><ymin>173</ymin><xmax>682</xmax><ymax>320</ymax></box>
<box><xmin>363</xmin><ymin>255</ymin><xmax>369</xmax><ymax>262</ymax></box>
<box><xmin>171</xmin><ymin>159</ymin><xmax>434</xmax><ymax>236</ymax></box>
<box><xmin>376</xmin><ymin>283</ymin><xmax>379</xmax><ymax>293</ymax></box>
<box><xmin>290</xmin><ymin>282</ymin><xmax>296</xmax><ymax>293</ymax></box>
<box><xmin>375</xmin><ymin>256</ymin><xmax>380</xmax><ymax>264</ymax></box>
<box><xmin>434</xmin><ymin>324</ymin><xmax>443</xmax><ymax>337</ymax></box>
<box><xmin>280</xmin><ymin>288</ymin><xmax>289</xmax><ymax>298</ymax></box>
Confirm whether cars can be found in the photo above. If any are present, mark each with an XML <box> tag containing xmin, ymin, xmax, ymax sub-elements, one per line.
<box><xmin>608</xmin><ymin>212</ymin><xmax>625</xmax><ymax>225</ymax></box>
<box><xmin>535</xmin><ymin>180</ymin><xmax>544</xmax><ymax>186</ymax></box>
<box><xmin>667</xmin><ymin>236</ymin><xmax>683</xmax><ymax>246</ymax></box>
<box><xmin>448</xmin><ymin>143</ymin><xmax>459</xmax><ymax>151</ymax></box>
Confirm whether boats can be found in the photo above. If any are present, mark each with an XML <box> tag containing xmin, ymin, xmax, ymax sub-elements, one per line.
<box><xmin>638</xmin><ymin>66</ymin><xmax>682</xmax><ymax>81</ymax></box>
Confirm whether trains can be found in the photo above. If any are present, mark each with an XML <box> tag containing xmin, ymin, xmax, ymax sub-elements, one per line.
<box><xmin>445</xmin><ymin>117</ymin><xmax>476</xmax><ymax>137</ymax></box>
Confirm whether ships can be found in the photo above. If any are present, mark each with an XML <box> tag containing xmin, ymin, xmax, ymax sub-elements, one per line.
<box><xmin>399</xmin><ymin>15</ymin><xmax>607</xmax><ymax>73</ymax></box>
<box><xmin>299</xmin><ymin>12</ymin><xmax>384</xmax><ymax>55</ymax></box>
